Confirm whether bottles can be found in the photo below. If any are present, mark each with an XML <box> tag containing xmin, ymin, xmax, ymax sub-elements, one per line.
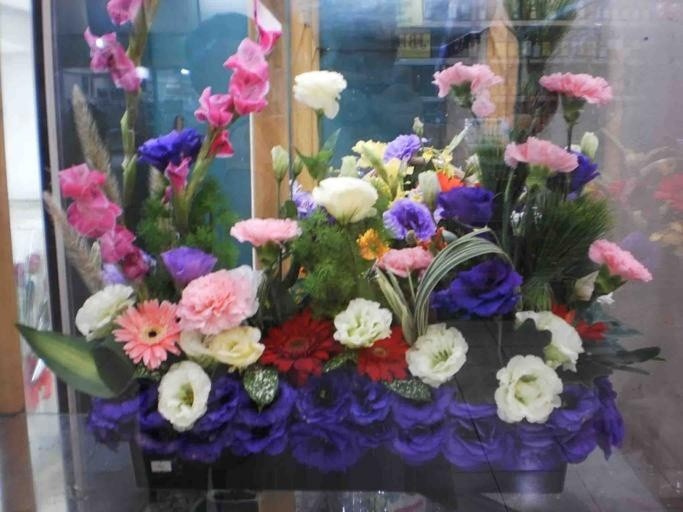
<box><xmin>435</xmin><ymin>1</ymin><xmax>654</xmax><ymax>95</ymax></box>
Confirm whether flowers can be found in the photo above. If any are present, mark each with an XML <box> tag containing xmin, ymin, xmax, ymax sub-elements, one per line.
<box><xmin>10</xmin><ymin>1</ymin><xmax>669</xmax><ymax>473</ymax></box>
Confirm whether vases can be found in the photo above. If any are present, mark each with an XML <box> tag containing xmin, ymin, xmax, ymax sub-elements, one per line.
<box><xmin>126</xmin><ymin>441</ymin><xmax>569</xmax><ymax>496</ymax></box>
